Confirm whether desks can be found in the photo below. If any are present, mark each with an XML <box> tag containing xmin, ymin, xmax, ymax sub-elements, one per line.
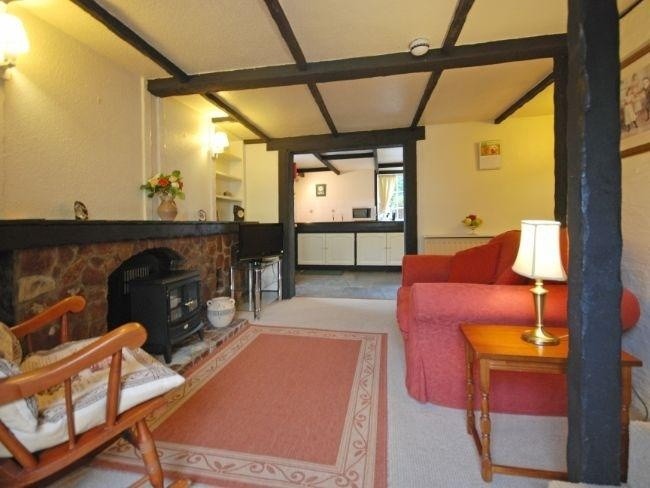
<box><xmin>228</xmin><ymin>258</ymin><xmax>282</xmax><ymax>321</ymax></box>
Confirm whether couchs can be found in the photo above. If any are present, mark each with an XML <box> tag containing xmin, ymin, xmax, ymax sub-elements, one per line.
<box><xmin>395</xmin><ymin>232</ymin><xmax>641</xmax><ymax>416</ymax></box>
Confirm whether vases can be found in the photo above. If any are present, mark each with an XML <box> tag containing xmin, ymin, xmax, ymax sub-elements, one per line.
<box><xmin>157</xmin><ymin>197</ymin><xmax>178</xmax><ymax>221</ymax></box>
<box><xmin>205</xmin><ymin>297</ymin><xmax>236</xmax><ymax>328</ymax></box>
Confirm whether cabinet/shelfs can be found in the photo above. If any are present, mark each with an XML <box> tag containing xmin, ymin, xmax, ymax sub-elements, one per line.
<box><xmin>213</xmin><ymin>124</ymin><xmax>244</xmax><ymax>222</ymax></box>
<box><xmin>297</xmin><ymin>232</ymin><xmax>356</xmax><ymax>268</ymax></box>
<box><xmin>356</xmin><ymin>231</ymin><xmax>405</xmax><ymax>269</ymax></box>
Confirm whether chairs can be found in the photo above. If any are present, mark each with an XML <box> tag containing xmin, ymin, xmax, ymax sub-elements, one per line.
<box><xmin>0</xmin><ymin>297</ymin><xmax>185</xmax><ymax>488</ymax></box>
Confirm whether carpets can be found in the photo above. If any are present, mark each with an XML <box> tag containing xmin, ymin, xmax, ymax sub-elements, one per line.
<box><xmin>79</xmin><ymin>319</ymin><xmax>387</xmax><ymax>488</ymax></box>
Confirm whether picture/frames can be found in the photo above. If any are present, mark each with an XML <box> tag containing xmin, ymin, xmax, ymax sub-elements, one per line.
<box><xmin>619</xmin><ymin>36</ymin><xmax>650</xmax><ymax>159</ymax></box>
<box><xmin>316</xmin><ymin>184</ymin><xmax>326</xmax><ymax>197</ymax></box>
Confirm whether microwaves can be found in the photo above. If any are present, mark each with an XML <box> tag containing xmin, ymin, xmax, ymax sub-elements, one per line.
<box><xmin>351</xmin><ymin>206</ymin><xmax>376</xmax><ymax>220</ymax></box>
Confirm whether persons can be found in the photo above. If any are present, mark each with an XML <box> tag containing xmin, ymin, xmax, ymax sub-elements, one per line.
<box><xmin>622</xmin><ymin>72</ymin><xmax>650</xmax><ymax>133</ymax></box>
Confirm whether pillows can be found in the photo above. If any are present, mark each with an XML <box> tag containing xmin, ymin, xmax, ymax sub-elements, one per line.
<box><xmin>448</xmin><ymin>243</ymin><xmax>502</xmax><ymax>284</ymax></box>
<box><xmin>495</xmin><ymin>263</ymin><xmax>525</xmax><ymax>285</ymax></box>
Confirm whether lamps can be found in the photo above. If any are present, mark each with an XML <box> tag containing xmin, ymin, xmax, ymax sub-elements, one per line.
<box><xmin>212</xmin><ymin>130</ymin><xmax>229</xmax><ymax>158</ymax></box>
<box><xmin>511</xmin><ymin>220</ymin><xmax>567</xmax><ymax>347</ymax></box>
<box><xmin>0</xmin><ymin>12</ymin><xmax>29</xmax><ymax>80</ymax></box>
<box><xmin>410</xmin><ymin>38</ymin><xmax>430</xmax><ymax>57</ymax></box>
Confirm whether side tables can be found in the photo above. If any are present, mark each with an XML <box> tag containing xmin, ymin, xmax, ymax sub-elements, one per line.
<box><xmin>459</xmin><ymin>324</ymin><xmax>642</xmax><ymax>483</ymax></box>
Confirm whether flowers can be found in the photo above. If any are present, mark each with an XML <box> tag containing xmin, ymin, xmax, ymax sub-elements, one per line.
<box><xmin>139</xmin><ymin>170</ymin><xmax>185</xmax><ymax>200</ymax></box>
